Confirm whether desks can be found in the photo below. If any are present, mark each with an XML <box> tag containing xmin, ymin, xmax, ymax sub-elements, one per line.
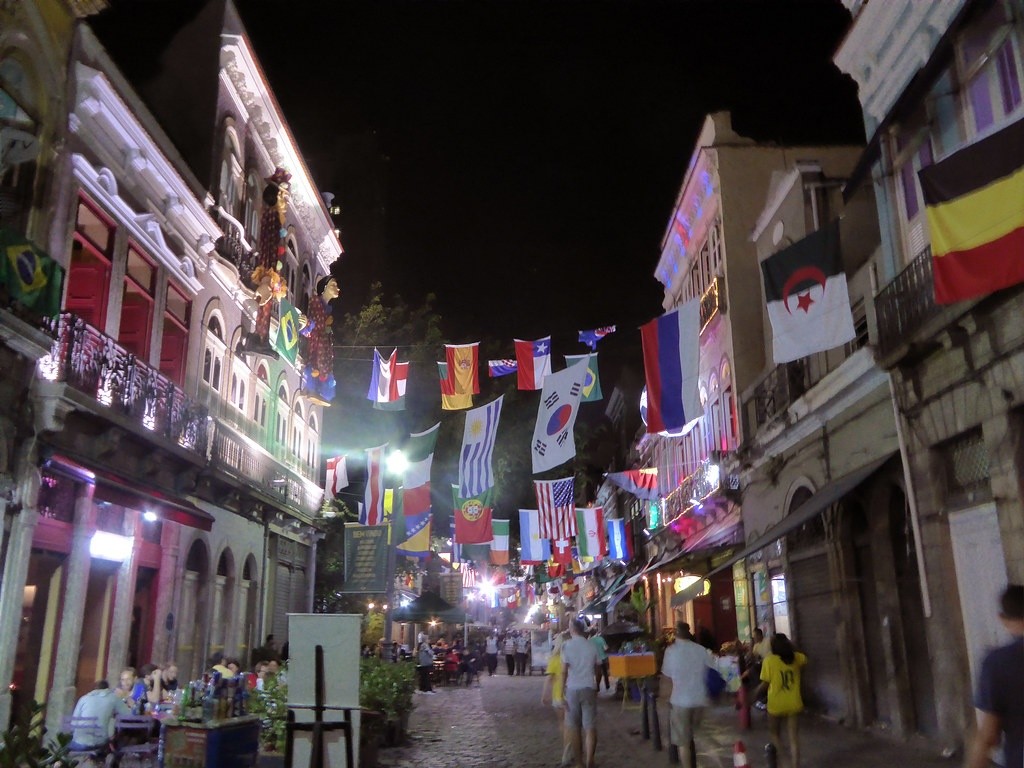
<box><xmin>718</xmin><ymin>655</ymin><xmax>741</xmax><ymax>694</ymax></box>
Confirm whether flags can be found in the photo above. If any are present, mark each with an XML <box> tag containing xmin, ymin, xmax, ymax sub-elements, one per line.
<box><xmin>531</xmin><ymin>356</ymin><xmax>590</xmax><ymax>474</ymax></box>
<box><xmin>564</xmin><ymin>352</ymin><xmax>603</xmax><ymax>401</ymax></box>
<box><xmin>0</xmin><ymin>222</ymin><xmax>62</xmax><ymax>319</ymax></box>
<box><xmin>918</xmin><ymin>117</ymin><xmax>1024</xmax><ymax>306</ymax></box>
<box><xmin>760</xmin><ymin>217</ymin><xmax>856</xmax><ymax>365</ymax></box>
<box><xmin>604</xmin><ymin>464</ymin><xmax>658</xmax><ymax>504</ymax></box>
<box><xmin>489</xmin><ymin>359</ymin><xmax>517</xmax><ymax>377</ymax></box>
<box><xmin>366</xmin><ymin>348</ymin><xmax>409</xmax><ymax>411</ymax></box>
<box><xmin>519</xmin><ymin>478</ymin><xmax>634</xmax><ymax>594</ymax></box>
<box><xmin>436</xmin><ymin>342</ymin><xmax>480</xmax><ymax>410</ymax></box>
<box><xmin>640</xmin><ymin>298</ymin><xmax>703</xmax><ymax>431</ymax></box>
<box><xmin>461</xmin><ymin>568</ymin><xmax>535</xmax><ymax>608</ymax></box>
<box><xmin>270</xmin><ymin>297</ymin><xmax>303</xmax><ymax>368</ymax></box>
<box><xmin>578</xmin><ymin>325</ymin><xmax>618</xmax><ymax>351</ymax></box>
<box><xmin>513</xmin><ymin>335</ymin><xmax>550</xmax><ymax>390</ymax></box>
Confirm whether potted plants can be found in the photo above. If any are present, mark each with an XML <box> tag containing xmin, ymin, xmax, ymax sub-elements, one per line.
<box><xmin>250</xmin><ymin>653</ymin><xmax>419</xmax><ymax>768</ymax></box>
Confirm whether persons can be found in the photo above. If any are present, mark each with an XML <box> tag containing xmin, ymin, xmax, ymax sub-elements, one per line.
<box><xmin>202</xmin><ymin>635</ymin><xmax>288</xmax><ymax>751</ymax></box>
<box><xmin>448</xmin><ymin>394</ymin><xmax>509</xmax><ymax>565</ymax></box>
<box><xmin>360</xmin><ymin>640</ymin><xmax>409</xmax><ymax>660</ymax></box>
<box><xmin>964</xmin><ymin>584</ymin><xmax>1024</xmax><ymax>768</ymax></box>
<box><xmin>357</xmin><ymin>421</ymin><xmax>442</xmax><ymax>557</ymax></box>
<box><xmin>661</xmin><ymin>621</ymin><xmax>723</xmax><ymax>768</ymax></box>
<box><xmin>48</xmin><ymin>664</ymin><xmax>178</xmax><ymax>765</ymax></box>
<box><xmin>415</xmin><ymin>629</ymin><xmax>531</xmax><ymax>695</ymax></box>
<box><xmin>752</xmin><ymin>629</ymin><xmax>809</xmax><ymax>768</ymax></box>
<box><xmin>323</xmin><ymin>456</ymin><xmax>349</xmax><ymax>499</ymax></box>
<box><xmin>540</xmin><ymin>617</ymin><xmax>610</xmax><ymax>768</ymax></box>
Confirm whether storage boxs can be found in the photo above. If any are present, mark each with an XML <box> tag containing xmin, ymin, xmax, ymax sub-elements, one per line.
<box><xmin>608</xmin><ymin>652</ymin><xmax>656</xmax><ymax>677</ymax></box>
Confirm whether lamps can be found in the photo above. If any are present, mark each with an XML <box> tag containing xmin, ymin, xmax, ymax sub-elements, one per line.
<box><xmin>619</xmin><ymin>559</ymin><xmax>626</xmax><ymax>565</ymax></box>
<box><xmin>643</xmin><ymin>527</ymin><xmax>652</xmax><ymax>535</ymax></box>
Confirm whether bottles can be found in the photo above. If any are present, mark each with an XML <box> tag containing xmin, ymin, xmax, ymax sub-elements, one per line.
<box><xmin>151</xmin><ymin>670</ymin><xmax>268</xmax><ymax>723</ymax></box>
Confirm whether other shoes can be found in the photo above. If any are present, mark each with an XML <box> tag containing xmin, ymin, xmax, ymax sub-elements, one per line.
<box><xmin>418</xmin><ymin>689</ymin><xmax>436</xmax><ymax>694</ymax></box>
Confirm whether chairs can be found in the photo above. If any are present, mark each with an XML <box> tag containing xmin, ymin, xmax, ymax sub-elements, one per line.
<box><xmin>429</xmin><ymin>651</ymin><xmax>482</xmax><ymax>687</ymax></box>
<box><xmin>61</xmin><ymin>703</ymin><xmax>173</xmax><ymax>768</ymax></box>
